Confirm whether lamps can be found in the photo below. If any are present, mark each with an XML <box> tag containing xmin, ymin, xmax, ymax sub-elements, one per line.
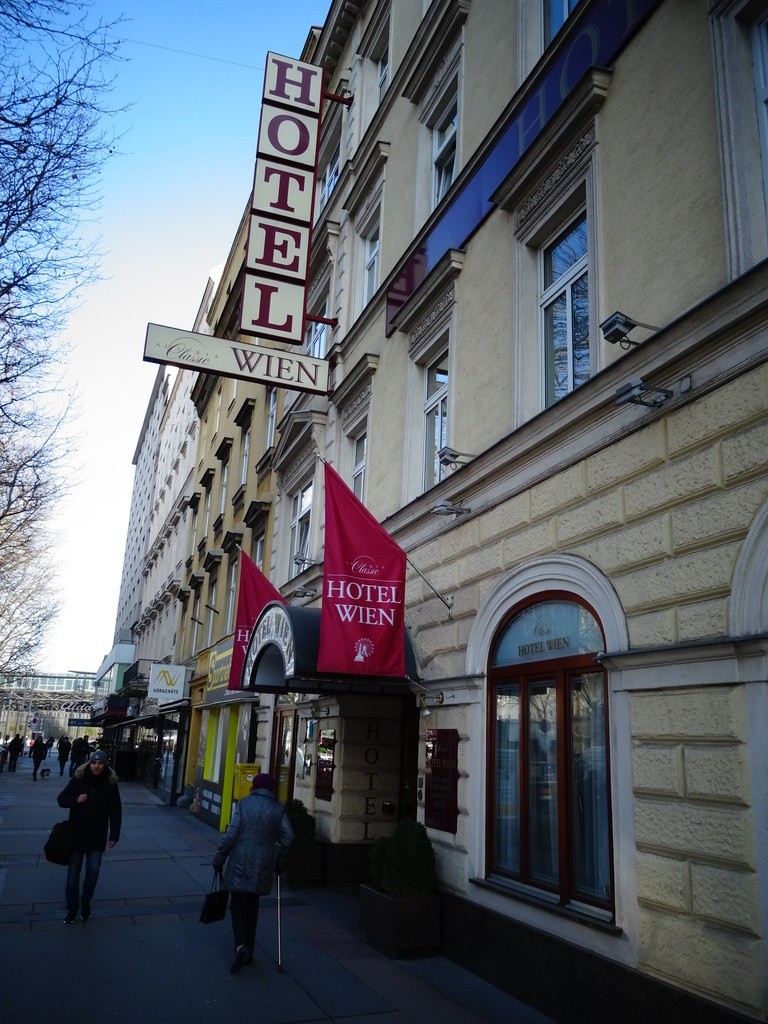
<box><xmin>601</xmin><ymin>311</ymin><xmax>662</xmax><ymax>348</ymax></box>
<box><xmin>614</xmin><ymin>379</ymin><xmax>672</xmax><ymax>409</ymax></box>
<box><xmin>292</xmin><ymin>585</ymin><xmax>318</xmax><ymax>599</ymax></box>
<box><xmin>438</xmin><ymin>446</ymin><xmax>477</xmax><ymax>467</ymax></box>
<box><xmin>294</xmin><ymin>554</ymin><xmax>321</xmax><ymax>568</ymax></box>
<box><xmin>431</xmin><ymin>500</ymin><xmax>471</xmax><ymax>518</ymax></box>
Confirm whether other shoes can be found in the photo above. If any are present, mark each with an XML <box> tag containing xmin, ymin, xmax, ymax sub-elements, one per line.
<box><xmin>230</xmin><ymin>952</ymin><xmax>252</xmax><ymax>973</ymax></box>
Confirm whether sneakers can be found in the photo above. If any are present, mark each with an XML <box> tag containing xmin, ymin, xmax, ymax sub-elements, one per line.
<box><xmin>80</xmin><ymin>901</ymin><xmax>92</xmax><ymax>921</ymax></box>
<box><xmin>63</xmin><ymin>910</ymin><xmax>80</xmax><ymax>923</ymax></box>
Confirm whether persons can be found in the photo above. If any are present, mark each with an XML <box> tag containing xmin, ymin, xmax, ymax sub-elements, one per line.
<box><xmin>212</xmin><ymin>773</ymin><xmax>296</xmax><ymax>974</ymax></box>
<box><xmin>0</xmin><ymin>732</ymin><xmax>96</xmax><ymax>782</ymax></box>
<box><xmin>56</xmin><ymin>751</ymin><xmax>124</xmax><ymax>925</ymax></box>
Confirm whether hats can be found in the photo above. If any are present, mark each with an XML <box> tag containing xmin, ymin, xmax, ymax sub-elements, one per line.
<box><xmin>253</xmin><ymin>773</ymin><xmax>273</xmax><ymax>789</ymax></box>
<box><xmin>91</xmin><ymin>751</ymin><xmax>108</xmax><ymax>762</ymax></box>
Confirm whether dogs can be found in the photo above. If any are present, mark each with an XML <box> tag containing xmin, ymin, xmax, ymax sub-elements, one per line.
<box><xmin>40</xmin><ymin>768</ymin><xmax>50</xmax><ymax>779</ymax></box>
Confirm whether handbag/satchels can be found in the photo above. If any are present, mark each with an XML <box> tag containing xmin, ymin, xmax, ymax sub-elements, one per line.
<box><xmin>43</xmin><ymin>820</ymin><xmax>73</xmax><ymax>865</ymax></box>
<box><xmin>199</xmin><ymin>870</ymin><xmax>229</xmax><ymax>925</ymax></box>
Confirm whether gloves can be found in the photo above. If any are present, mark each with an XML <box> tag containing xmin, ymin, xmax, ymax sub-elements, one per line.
<box><xmin>212</xmin><ymin>854</ymin><xmax>227</xmax><ymax>872</ymax></box>
<box><xmin>274</xmin><ymin>846</ymin><xmax>291</xmax><ymax>875</ymax></box>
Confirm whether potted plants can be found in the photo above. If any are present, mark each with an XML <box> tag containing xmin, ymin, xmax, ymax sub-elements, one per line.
<box><xmin>273</xmin><ymin>797</ymin><xmax>324</xmax><ymax>890</ymax></box>
<box><xmin>354</xmin><ymin>818</ymin><xmax>446</xmax><ymax>959</ymax></box>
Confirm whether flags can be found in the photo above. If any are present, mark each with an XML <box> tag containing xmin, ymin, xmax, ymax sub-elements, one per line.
<box><xmin>227</xmin><ymin>548</ymin><xmax>288</xmax><ymax>692</ymax></box>
<box><xmin>318</xmin><ymin>462</ymin><xmax>406</xmax><ymax>677</ymax></box>
